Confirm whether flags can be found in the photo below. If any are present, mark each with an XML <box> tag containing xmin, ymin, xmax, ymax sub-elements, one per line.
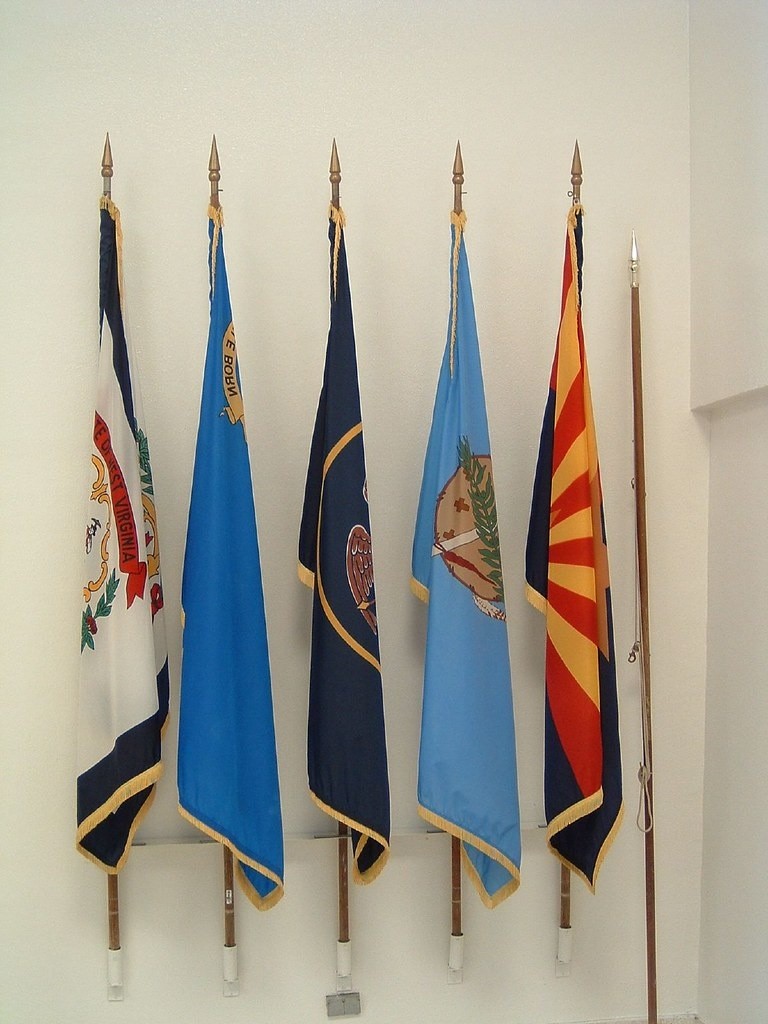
<box><xmin>409</xmin><ymin>210</ymin><xmax>524</xmax><ymax>910</ymax></box>
<box><xmin>176</xmin><ymin>203</ymin><xmax>285</xmax><ymax>913</ymax></box>
<box><xmin>74</xmin><ymin>192</ymin><xmax>170</xmax><ymax>876</ymax></box>
<box><xmin>297</xmin><ymin>204</ymin><xmax>392</xmax><ymax>887</ymax></box>
<box><xmin>524</xmin><ymin>205</ymin><xmax>625</xmax><ymax>894</ymax></box>
